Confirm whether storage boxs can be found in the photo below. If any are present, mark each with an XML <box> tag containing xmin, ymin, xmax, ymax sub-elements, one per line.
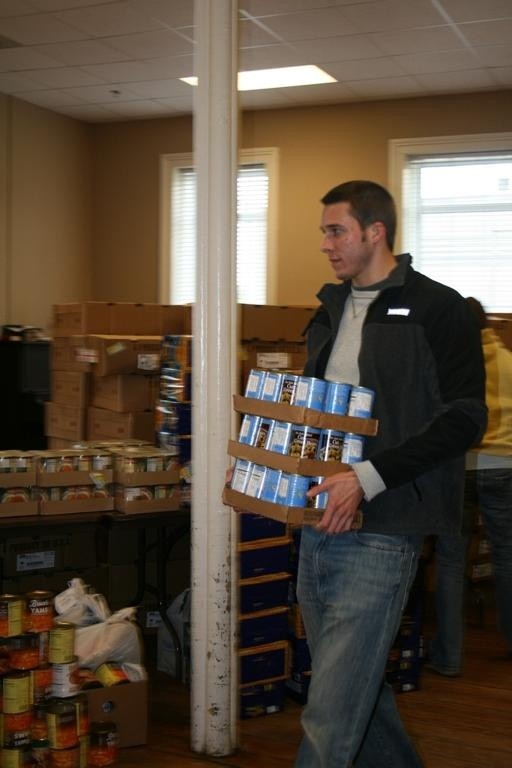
<box><xmin>80</xmin><ymin>680</ymin><xmax>148</xmax><ymax>750</ymax></box>
<box><xmin>42</xmin><ymin>300</ymin><xmax>320</xmax><ymax>447</ymax></box>
<box><xmin>0</xmin><ymin>509</ymin><xmax>192</xmax><ymax>667</ymax></box>
<box><xmin>237</xmin><ymin>513</ymin><xmax>422</xmax><ymax>719</ymax></box>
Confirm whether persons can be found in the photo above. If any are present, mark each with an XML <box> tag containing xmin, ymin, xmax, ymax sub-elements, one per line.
<box><xmin>424</xmin><ymin>296</ymin><xmax>512</xmax><ymax>678</ymax></box>
<box><xmin>221</xmin><ymin>179</ymin><xmax>489</xmax><ymax>767</ymax></box>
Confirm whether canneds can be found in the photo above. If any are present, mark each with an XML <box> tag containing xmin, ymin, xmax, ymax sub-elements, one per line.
<box><xmin>0</xmin><ymin>591</ymin><xmax>130</xmax><ymax>768</ymax></box>
<box><xmin>230</xmin><ymin>367</ymin><xmax>374</xmax><ymax>511</ymax></box>
<box><xmin>0</xmin><ymin>438</ymin><xmax>179</xmax><ymax>501</ymax></box>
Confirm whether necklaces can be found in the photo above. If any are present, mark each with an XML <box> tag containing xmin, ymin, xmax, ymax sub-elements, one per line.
<box><xmin>351</xmin><ymin>295</ymin><xmax>372</xmax><ymax>319</ymax></box>
